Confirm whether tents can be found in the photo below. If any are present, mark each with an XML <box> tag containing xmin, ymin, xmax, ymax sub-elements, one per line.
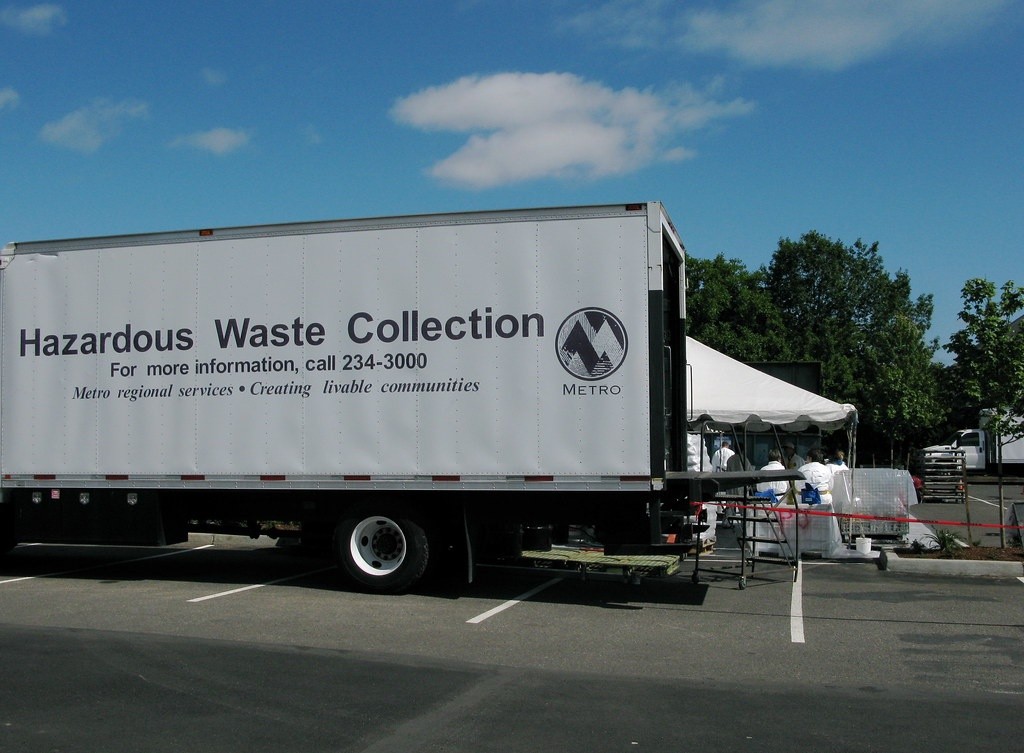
<box><xmin>685</xmin><ymin>333</ymin><xmax>859</xmax><ymax>550</ymax></box>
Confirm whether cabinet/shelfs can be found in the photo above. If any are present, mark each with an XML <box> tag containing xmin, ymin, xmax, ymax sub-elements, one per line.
<box><xmin>920</xmin><ymin>449</ymin><xmax>965</xmax><ymax>503</ymax></box>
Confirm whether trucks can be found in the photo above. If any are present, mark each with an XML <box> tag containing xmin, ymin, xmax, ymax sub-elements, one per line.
<box><xmin>0</xmin><ymin>202</ymin><xmax>806</xmax><ymax>606</ymax></box>
<box><xmin>922</xmin><ymin>408</ymin><xmax>1024</xmax><ymax>475</ymax></box>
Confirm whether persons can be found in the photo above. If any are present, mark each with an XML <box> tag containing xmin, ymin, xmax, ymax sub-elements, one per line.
<box><xmin>784</xmin><ymin>443</ymin><xmax>805</xmax><ymax>470</ymax></box>
<box><xmin>912</xmin><ymin>474</ymin><xmax>923</xmax><ymax>502</ymax></box>
<box><xmin>757</xmin><ymin>449</ymin><xmax>791</xmax><ymax>503</ymax></box>
<box><xmin>712</xmin><ymin>439</ymin><xmax>735</xmax><ymax>468</ymax></box>
<box><xmin>825</xmin><ymin>446</ymin><xmax>850</xmax><ymax>471</ymax></box>
<box><xmin>794</xmin><ymin>448</ymin><xmax>834</xmax><ymax>504</ymax></box>
